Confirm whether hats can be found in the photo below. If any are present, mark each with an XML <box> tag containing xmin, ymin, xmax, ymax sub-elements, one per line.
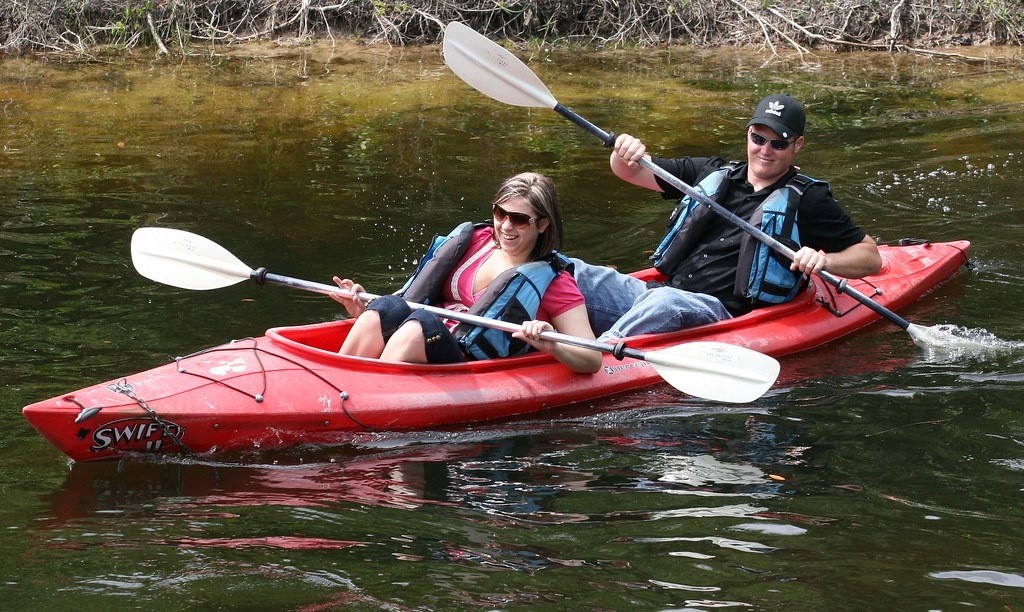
<box><xmin>745</xmin><ymin>93</ymin><xmax>806</xmax><ymax>140</ymax></box>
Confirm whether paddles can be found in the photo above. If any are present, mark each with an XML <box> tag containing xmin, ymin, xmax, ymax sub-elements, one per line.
<box><xmin>126</xmin><ymin>223</ymin><xmax>783</xmax><ymax>404</ymax></box>
<box><xmin>438</xmin><ymin>21</ymin><xmax>982</xmax><ymax>355</ymax></box>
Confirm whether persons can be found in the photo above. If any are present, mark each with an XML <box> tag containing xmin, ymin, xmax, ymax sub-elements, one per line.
<box><xmin>573</xmin><ymin>92</ymin><xmax>884</xmax><ymax>347</ymax></box>
<box><xmin>330</xmin><ymin>171</ymin><xmax>603</xmax><ymax>375</ymax></box>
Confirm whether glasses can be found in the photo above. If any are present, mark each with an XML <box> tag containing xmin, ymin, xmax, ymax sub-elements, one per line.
<box><xmin>749</xmin><ymin>126</ymin><xmax>796</xmax><ymax>151</ymax></box>
<box><xmin>489</xmin><ymin>202</ymin><xmax>542</xmax><ymax>229</ymax></box>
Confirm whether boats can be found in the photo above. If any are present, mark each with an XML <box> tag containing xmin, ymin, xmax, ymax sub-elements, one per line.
<box><xmin>17</xmin><ymin>238</ymin><xmax>975</xmax><ymax>464</ymax></box>
